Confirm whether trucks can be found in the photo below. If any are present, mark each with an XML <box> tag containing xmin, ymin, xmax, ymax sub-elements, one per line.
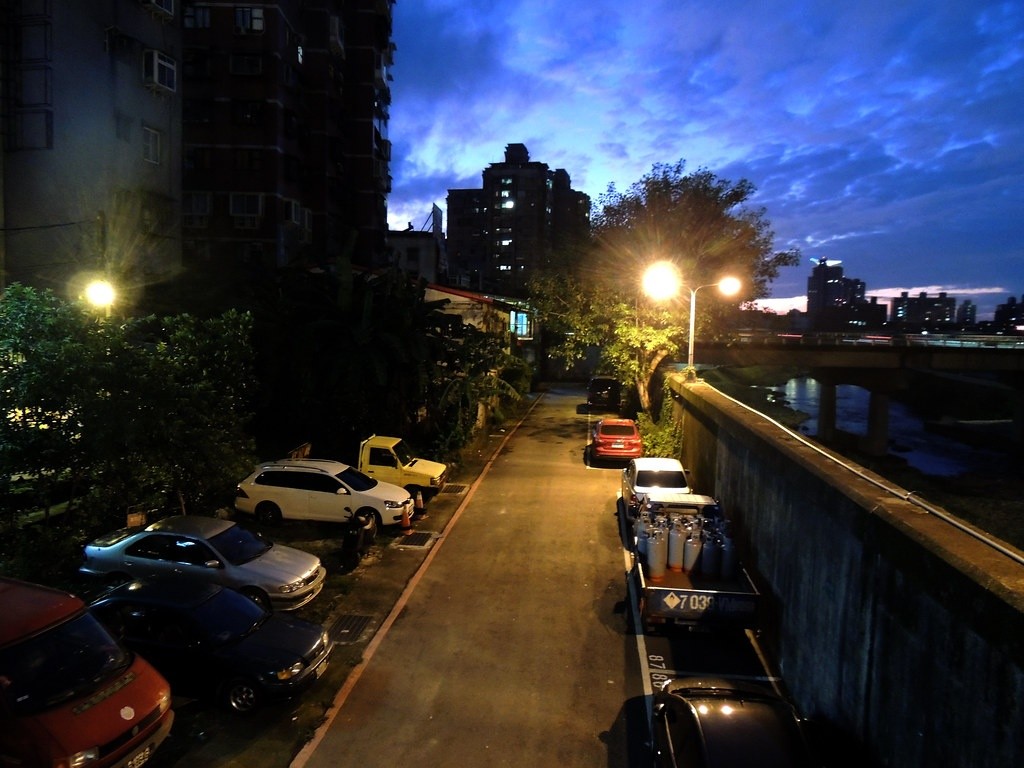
<box><xmin>357</xmin><ymin>433</ymin><xmax>447</xmax><ymax>504</ymax></box>
<box><xmin>632</xmin><ymin>491</ymin><xmax>763</xmax><ymax>636</ymax></box>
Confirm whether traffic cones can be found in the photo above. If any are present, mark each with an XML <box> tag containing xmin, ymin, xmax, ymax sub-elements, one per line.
<box><xmin>392</xmin><ymin>504</ymin><xmax>415</xmax><ymax>537</ymax></box>
<box><xmin>414</xmin><ymin>491</ymin><xmax>430</xmax><ymax>520</ymax></box>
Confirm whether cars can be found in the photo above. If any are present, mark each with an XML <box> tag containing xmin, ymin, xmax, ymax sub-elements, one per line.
<box><xmin>80</xmin><ymin>513</ymin><xmax>327</xmax><ymax>618</ymax></box>
<box><xmin>644</xmin><ymin>673</ymin><xmax>820</xmax><ymax>768</ymax></box>
<box><xmin>84</xmin><ymin>573</ymin><xmax>336</xmax><ymax>719</ymax></box>
<box><xmin>231</xmin><ymin>457</ymin><xmax>416</xmax><ymax>541</ymax></box>
<box><xmin>584</xmin><ymin>374</ymin><xmax>621</xmax><ymax>412</ymax></box>
<box><xmin>589</xmin><ymin>417</ymin><xmax>644</xmax><ymax>463</ymax></box>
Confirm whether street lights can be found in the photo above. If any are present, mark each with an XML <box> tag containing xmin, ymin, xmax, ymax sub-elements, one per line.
<box><xmin>643</xmin><ymin>259</ymin><xmax>743</xmax><ymax>370</ymax></box>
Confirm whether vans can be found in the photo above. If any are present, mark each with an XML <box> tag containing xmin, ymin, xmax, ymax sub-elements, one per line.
<box><xmin>620</xmin><ymin>456</ymin><xmax>693</xmax><ymax>525</ymax></box>
<box><xmin>0</xmin><ymin>578</ymin><xmax>179</xmax><ymax>768</ymax></box>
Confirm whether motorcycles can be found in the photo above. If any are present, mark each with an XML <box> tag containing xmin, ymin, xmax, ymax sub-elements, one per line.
<box><xmin>333</xmin><ymin>505</ymin><xmax>382</xmax><ymax>572</ymax></box>
